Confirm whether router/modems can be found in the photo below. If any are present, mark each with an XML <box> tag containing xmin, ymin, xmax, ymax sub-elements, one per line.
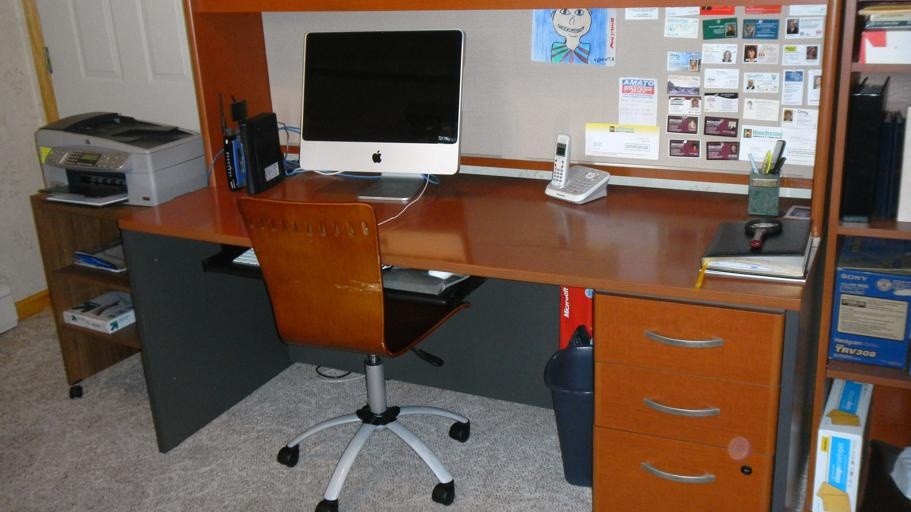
<box><xmin>223</xmin><ymin>132</ymin><xmax>245</xmax><ymax>192</ymax></box>
<box><xmin>241</xmin><ymin>112</ymin><xmax>286</xmax><ymax>195</ymax></box>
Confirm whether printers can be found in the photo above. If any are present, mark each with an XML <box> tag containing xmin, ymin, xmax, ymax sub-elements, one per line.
<box><xmin>34</xmin><ymin>112</ymin><xmax>207</xmax><ymax>208</ymax></box>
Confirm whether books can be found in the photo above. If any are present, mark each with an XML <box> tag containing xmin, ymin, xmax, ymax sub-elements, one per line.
<box><xmin>701</xmin><ymin>217</ymin><xmax>815</xmax><ymax>280</ymax></box>
<box><xmin>836</xmin><ymin>2</ymin><xmax>911</xmax><ymax>226</ymax></box>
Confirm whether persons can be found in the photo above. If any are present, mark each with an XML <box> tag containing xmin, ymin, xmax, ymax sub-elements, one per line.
<box><xmin>725</xmin><ymin>24</ymin><xmax>736</xmax><ymax>37</ymax></box>
<box><xmin>723</xmin><ymin>51</ymin><xmax>733</xmax><ymax>62</ymax></box>
<box><xmin>814</xmin><ymin>76</ymin><xmax>822</xmax><ymax>89</ymax></box>
<box><xmin>788</xmin><ymin>19</ymin><xmax>800</xmax><ymax>34</ymax></box>
<box><xmin>689</xmin><ymin>60</ymin><xmax>698</xmax><ymax>73</ymax></box>
<box><xmin>747</xmin><ymin>100</ymin><xmax>754</xmax><ymax>110</ymax></box>
<box><xmin>746</xmin><ymin>80</ymin><xmax>756</xmax><ymax>89</ymax></box>
<box><xmin>691</xmin><ymin>145</ymin><xmax>698</xmax><ymax>154</ymax></box>
<box><xmin>745</xmin><ymin>46</ymin><xmax>758</xmax><ymax>63</ymax></box>
<box><xmin>746</xmin><ymin>25</ymin><xmax>756</xmax><ymax>39</ymax></box>
<box><xmin>784</xmin><ymin>110</ymin><xmax>794</xmax><ymax>122</ymax></box>
<box><xmin>692</xmin><ymin>98</ymin><xmax>701</xmax><ymax>108</ymax></box>
<box><xmin>808</xmin><ymin>47</ymin><xmax>818</xmax><ymax>60</ymax></box>
<box><xmin>730</xmin><ymin>145</ymin><xmax>737</xmax><ymax>155</ymax></box>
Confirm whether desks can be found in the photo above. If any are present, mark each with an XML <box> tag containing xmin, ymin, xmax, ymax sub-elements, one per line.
<box><xmin>120</xmin><ymin>174</ymin><xmax>815</xmax><ymax>511</ymax></box>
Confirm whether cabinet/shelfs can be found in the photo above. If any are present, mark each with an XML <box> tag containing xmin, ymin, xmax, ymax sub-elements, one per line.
<box><xmin>30</xmin><ymin>188</ymin><xmax>150</xmax><ymax>398</ymax></box>
<box><xmin>803</xmin><ymin>0</ymin><xmax>910</xmax><ymax>512</ymax></box>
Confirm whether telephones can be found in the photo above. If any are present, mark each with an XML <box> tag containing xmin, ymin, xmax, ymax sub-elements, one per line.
<box><xmin>544</xmin><ymin>133</ymin><xmax>610</xmax><ymax>205</ymax></box>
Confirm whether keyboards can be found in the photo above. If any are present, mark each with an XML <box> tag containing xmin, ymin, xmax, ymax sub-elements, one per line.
<box><xmin>233</xmin><ymin>247</ymin><xmax>259</xmax><ymax>268</ymax></box>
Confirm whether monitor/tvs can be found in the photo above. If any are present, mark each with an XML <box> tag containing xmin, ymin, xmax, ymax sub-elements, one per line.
<box><xmin>299</xmin><ymin>29</ymin><xmax>465</xmax><ymax>201</ymax></box>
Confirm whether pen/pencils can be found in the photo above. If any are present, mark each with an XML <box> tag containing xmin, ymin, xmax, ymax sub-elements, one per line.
<box><xmin>748</xmin><ymin>153</ymin><xmax>786</xmax><ymax>174</ymax></box>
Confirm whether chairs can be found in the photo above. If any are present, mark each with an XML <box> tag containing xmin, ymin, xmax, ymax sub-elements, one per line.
<box><xmin>237</xmin><ymin>195</ymin><xmax>469</xmax><ymax>512</ymax></box>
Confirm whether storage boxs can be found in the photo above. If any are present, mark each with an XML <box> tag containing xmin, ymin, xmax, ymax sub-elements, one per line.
<box><xmin>827</xmin><ymin>241</ymin><xmax>911</xmax><ymax>374</ymax></box>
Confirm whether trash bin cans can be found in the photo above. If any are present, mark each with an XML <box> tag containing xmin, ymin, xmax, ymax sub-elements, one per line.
<box><xmin>545</xmin><ymin>345</ymin><xmax>592</xmax><ymax>486</ymax></box>
<box><xmin>861</xmin><ymin>439</ymin><xmax>910</xmax><ymax>511</ymax></box>
